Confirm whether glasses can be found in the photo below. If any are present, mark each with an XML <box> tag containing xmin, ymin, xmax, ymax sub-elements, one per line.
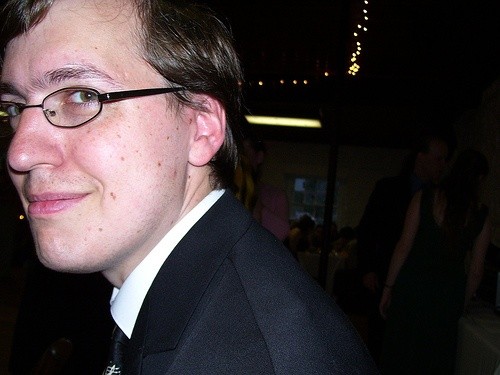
<box><xmin>0</xmin><ymin>87</ymin><xmax>193</xmax><ymax>138</ymax></box>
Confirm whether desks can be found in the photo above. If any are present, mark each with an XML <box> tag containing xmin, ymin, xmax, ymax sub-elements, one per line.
<box><xmin>456</xmin><ymin>309</ymin><xmax>500</xmax><ymax>375</ymax></box>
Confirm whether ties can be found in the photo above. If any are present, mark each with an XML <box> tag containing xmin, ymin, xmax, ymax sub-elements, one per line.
<box><xmin>100</xmin><ymin>325</ymin><xmax>129</xmax><ymax>375</ymax></box>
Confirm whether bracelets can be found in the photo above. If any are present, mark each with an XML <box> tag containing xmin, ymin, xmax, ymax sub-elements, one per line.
<box><xmin>383</xmin><ymin>284</ymin><xmax>392</xmax><ymax>289</ymax></box>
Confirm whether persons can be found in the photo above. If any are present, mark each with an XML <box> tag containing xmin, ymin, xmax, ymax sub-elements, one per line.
<box><xmin>0</xmin><ymin>0</ymin><xmax>369</xmax><ymax>375</ymax></box>
<box><xmin>234</xmin><ymin>125</ymin><xmax>500</xmax><ymax>375</ymax></box>
